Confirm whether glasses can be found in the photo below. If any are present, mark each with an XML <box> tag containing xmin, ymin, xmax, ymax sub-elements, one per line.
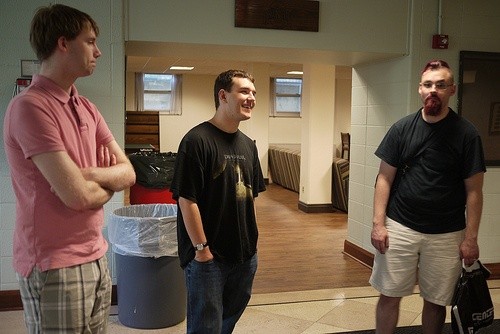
<box><xmin>418</xmin><ymin>80</ymin><xmax>453</xmax><ymax>92</ymax></box>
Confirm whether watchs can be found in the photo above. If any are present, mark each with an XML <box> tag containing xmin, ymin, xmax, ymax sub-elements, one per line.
<box><xmin>193</xmin><ymin>241</ymin><xmax>209</xmax><ymax>251</ymax></box>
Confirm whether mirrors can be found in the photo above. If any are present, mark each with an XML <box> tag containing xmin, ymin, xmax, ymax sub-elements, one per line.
<box><xmin>457</xmin><ymin>50</ymin><xmax>500</xmax><ymax>167</ymax></box>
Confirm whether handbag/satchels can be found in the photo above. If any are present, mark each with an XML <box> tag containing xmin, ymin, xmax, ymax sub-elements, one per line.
<box><xmin>451</xmin><ymin>256</ymin><xmax>494</xmax><ymax>334</ymax></box>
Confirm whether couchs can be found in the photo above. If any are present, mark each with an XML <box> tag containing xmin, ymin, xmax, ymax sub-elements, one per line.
<box><xmin>267</xmin><ymin>147</ymin><xmax>350</xmax><ymax>214</ymax></box>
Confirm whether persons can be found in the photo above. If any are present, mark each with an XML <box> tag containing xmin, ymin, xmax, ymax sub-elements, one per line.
<box><xmin>170</xmin><ymin>70</ymin><xmax>266</xmax><ymax>334</ymax></box>
<box><xmin>4</xmin><ymin>2</ymin><xmax>138</xmax><ymax>334</ymax></box>
<box><xmin>370</xmin><ymin>61</ymin><xmax>486</xmax><ymax>334</ymax></box>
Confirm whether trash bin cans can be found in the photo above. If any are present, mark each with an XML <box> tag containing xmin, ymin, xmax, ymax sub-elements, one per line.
<box><xmin>127</xmin><ymin>150</ymin><xmax>178</xmax><ymax>205</ymax></box>
<box><xmin>110</xmin><ymin>205</ymin><xmax>187</xmax><ymax>329</ymax></box>
